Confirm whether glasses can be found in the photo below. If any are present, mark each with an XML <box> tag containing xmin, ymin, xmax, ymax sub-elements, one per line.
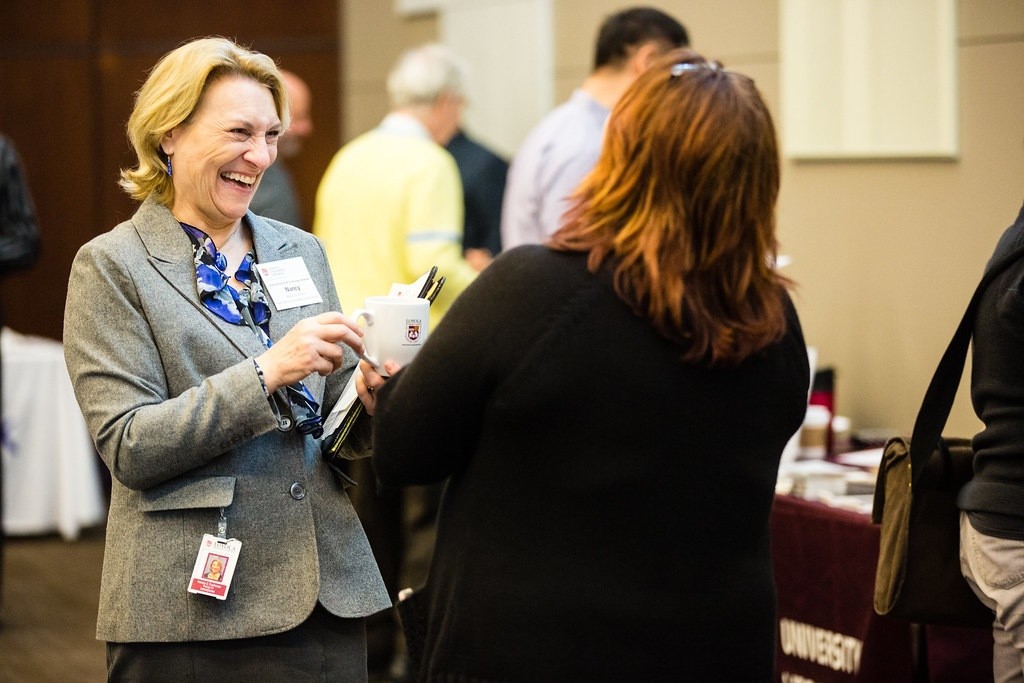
<box><xmin>667</xmin><ymin>62</ymin><xmax>756</xmax><ymax>86</ymax></box>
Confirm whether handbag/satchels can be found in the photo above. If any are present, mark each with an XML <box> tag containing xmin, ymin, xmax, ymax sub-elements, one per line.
<box><xmin>872</xmin><ymin>435</ymin><xmax>991</xmax><ymax>626</ymax></box>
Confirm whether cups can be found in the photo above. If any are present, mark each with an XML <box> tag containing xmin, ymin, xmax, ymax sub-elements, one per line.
<box><xmin>349</xmin><ymin>295</ymin><xmax>430</xmax><ymax>376</ymax></box>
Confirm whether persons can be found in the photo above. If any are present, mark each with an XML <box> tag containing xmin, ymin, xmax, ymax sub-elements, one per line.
<box><xmin>204</xmin><ymin>559</ymin><xmax>222</xmax><ymax>580</ymax></box>
<box><xmin>500</xmin><ymin>7</ymin><xmax>692</xmax><ymax>257</ymax></box>
<box><xmin>375</xmin><ymin>45</ymin><xmax>812</xmax><ymax>683</ymax></box>
<box><xmin>314</xmin><ymin>39</ymin><xmax>509</xmax><ymax>336</ymax></box>
<box><xmin>957</xmin><ymin>204</ymin><xmax>1024</xmax><ymax>683</ymax></box>
<box><xmin>0</xmin><ymin>130</ymin><xmax>45</xmax><ymax>329</ymax></box>
<box><xmin>64</xmin><ymin>35</ymin><xmax>401</xmax><ymax>683</ymax></box>
<box><xmin>251</xmin><ymin>63</ymin><xmax>311</xmax><ymax>230</ymax></box>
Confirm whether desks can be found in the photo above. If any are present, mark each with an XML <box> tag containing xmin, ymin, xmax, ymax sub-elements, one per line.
<box><xmin>768</xmin><ymin>443</ymin><xmax>972</xmax><ymax>683</ymax></box>
